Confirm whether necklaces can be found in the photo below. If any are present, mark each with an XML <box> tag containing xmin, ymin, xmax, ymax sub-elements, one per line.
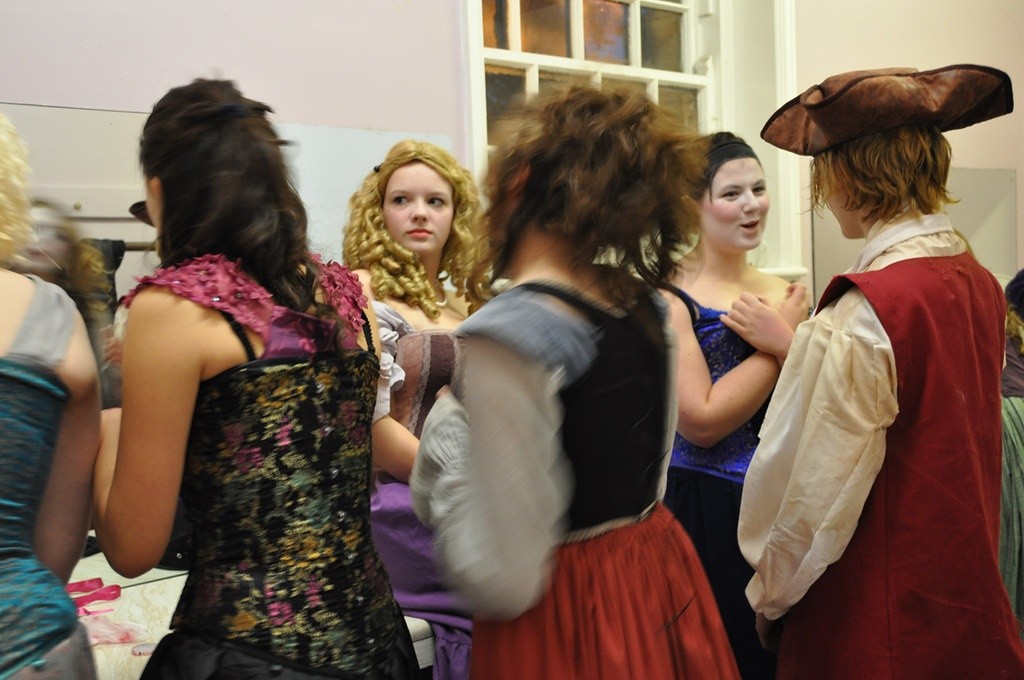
<box><xmin>435</xmin><ymin>289</ymin><xmax>448</xmax><ymax>307</ymax></box>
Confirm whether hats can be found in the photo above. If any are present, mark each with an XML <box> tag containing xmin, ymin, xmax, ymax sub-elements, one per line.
<box><xmin>759</xmin><ymin>63</ymin><xmax>1015</xmax><ymax>157</ymax></box>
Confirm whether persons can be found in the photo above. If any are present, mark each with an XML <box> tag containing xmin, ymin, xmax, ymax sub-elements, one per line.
<box><xmin>344</xmin><ymin>80</ymin><xmax>809</xmax><ymax>680</ymax></box>
<box><xmin>738</xmin><ymin>72</ymin><xmax>1024</xmax><ymax>679</ymax></box>
<box><xmin>0</xmin><ymin>117</ymin><xmax>121</xmax><ymax>680</ymax></box>
<box><xmin>85</xmin><ymin>79</ymin><xmax>420</xmax><ymax>679</ymax></box>
